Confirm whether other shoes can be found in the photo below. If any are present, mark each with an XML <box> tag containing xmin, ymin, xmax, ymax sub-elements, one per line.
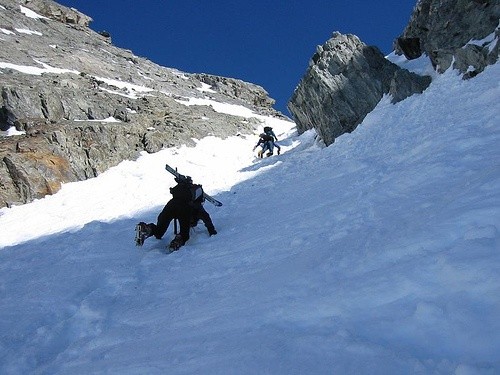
<box><xmin>134</xmin><ymin>221</ymin><xmax>151</xmax><ymax>246</ymax></box>
<box><xmin>169</xmin><ymin>238</ymin><xmax>184</xmax><ymax>253</ymax></box>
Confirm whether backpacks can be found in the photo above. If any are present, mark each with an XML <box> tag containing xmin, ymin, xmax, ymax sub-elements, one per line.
<box><xmin>170</xmin><ymin>183</ymin><xmax>203</xmax><ymax>200</ymax></box>
<box><xmin>264</xmin><ymin>136</ymin><xmax>274</xmax><ymax>141</ymax></box>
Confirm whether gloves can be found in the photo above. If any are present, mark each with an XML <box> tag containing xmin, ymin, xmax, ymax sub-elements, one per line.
<box><xmin>209</xmin><ymin>230</ymin><xmax>217</xmax><ymax>236</ymax></box>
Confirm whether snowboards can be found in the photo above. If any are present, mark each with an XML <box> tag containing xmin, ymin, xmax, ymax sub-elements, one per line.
<box><xmin>165</xmin><ymin>164</ymin><xmax>223</xmax><ymax>208</ymax></box>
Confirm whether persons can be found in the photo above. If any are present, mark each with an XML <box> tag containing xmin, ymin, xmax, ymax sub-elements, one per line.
<box><xmin>252</xmin><ymin>126</ymin><xmax>281</xmax><ymax>158</ymax></box>
<box><xmin>135</xmin><ymin>193</ymin><xmax>217</xmax><ymax>252</ymax></box>
